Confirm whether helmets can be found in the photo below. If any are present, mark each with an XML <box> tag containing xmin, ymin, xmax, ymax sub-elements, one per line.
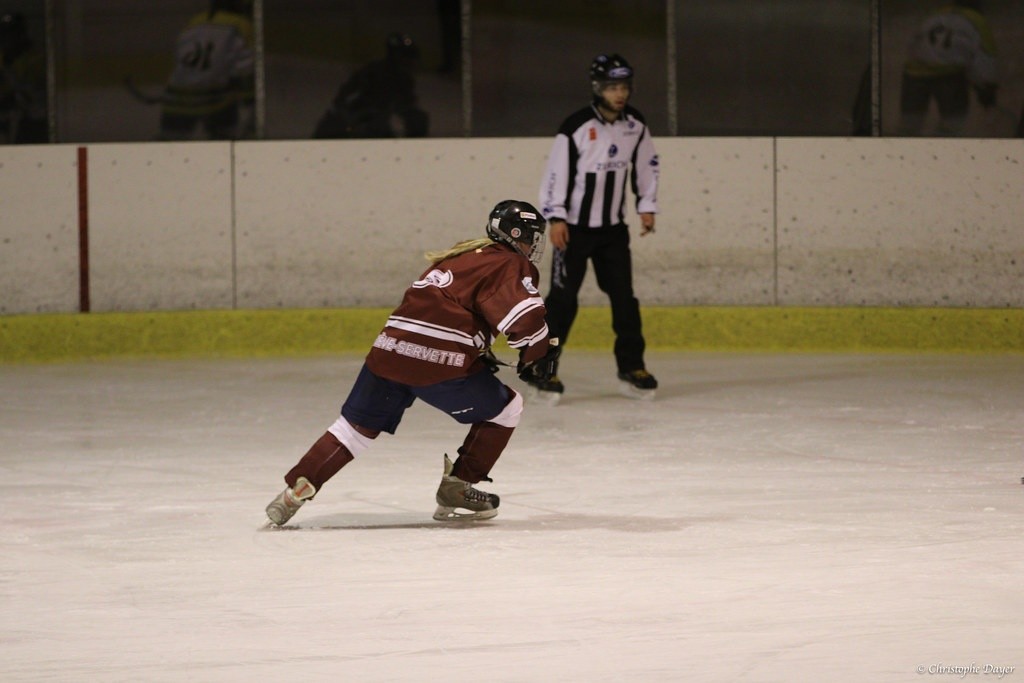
<box><xmin>486</xmin><ymin>200</ymin><xmax>547</xmax><ymax>252</ymax></box>
<box><xmin>590</xmin><ymin>53</ymin><xmax>634</xmax><ymax>82</ymax></box>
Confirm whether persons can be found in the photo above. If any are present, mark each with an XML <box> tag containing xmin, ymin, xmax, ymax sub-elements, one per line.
<box><xmin>262</xmin><ymin>198</ymin><xmax>556</xmax><ymax>531</ymax></box>
<box><xmin>309</xmin><ymin>31</ymin><xmax>427</xmax><ymax>138</ymax></box>
<box><xmin>527</xmin><ymin>52</ymin><xmax>660</xmax><ymax>406</ymax></box>
<box><xmin>897</xmin><ymin>0</ymin><xmax>998</xmax><ymax>138</ymax></box>
<box><xmin>156</xmin><ymin>0</ymin><xmax>254</xmax><ymax>140</ymax></box>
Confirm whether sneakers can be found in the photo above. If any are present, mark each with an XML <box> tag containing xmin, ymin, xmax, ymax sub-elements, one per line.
<box><xmin>527</xmin><ymin>376</ymin><xmax>565</xmax><ymax>406</ymax></box>
<box><xmin>618</xmin><ymin>370</ymin><xmax>658</xmax><ymax>400</ymax></box>
<box><xmin>431</xmin><ymin>453</ymin><xmax>500</xmax><ymax>521</ymax></box>
<box><xmin>266</xmin><ymin>477</ymin><xmax>316</xmax><ymax>526</ymax></box>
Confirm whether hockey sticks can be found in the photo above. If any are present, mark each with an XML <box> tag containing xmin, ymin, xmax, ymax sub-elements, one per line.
<box><xmin>495</xmin><ymin>358</ymin><xmax>558</xmax><ymax>376</ymax></box>
